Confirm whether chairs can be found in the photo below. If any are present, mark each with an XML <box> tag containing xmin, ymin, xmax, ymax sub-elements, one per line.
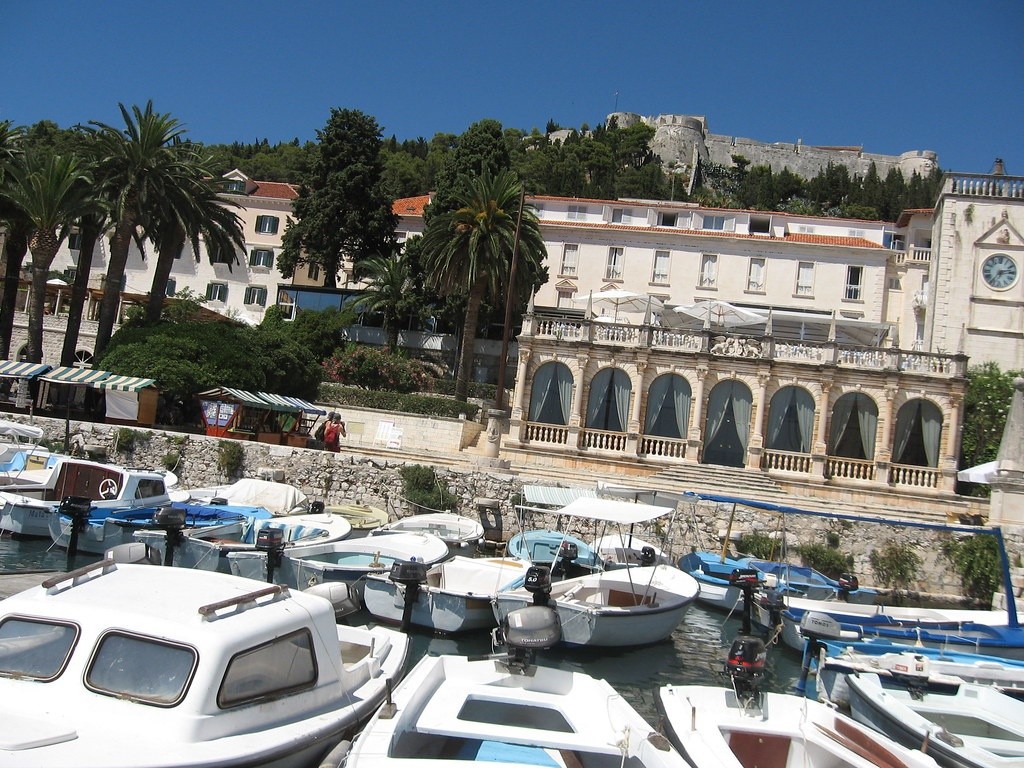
<box><xmin>946</xmin><ymin>510</ymin><xmax>988</xmax><ymax>526</ymax></box>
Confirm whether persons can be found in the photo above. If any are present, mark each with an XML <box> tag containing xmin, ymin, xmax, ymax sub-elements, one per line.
<box><xmin>320</xmin><ymin>411</ymin><xmax>346</xmax><ymax>453</ymax></box>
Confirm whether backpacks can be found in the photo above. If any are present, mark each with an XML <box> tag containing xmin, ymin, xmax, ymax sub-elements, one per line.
<box><xmin>324</xmin><ymin>423</ymin><xmax>340</xmax><ymax>448</ymax></box>
<box><xmin>315</xmin><ymin>420</ymin><xmax>331</xmax><ymax>441</ymax></box>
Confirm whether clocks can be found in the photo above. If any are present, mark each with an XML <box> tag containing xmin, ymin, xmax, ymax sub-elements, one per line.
<box><xmin>980</xmin><ymin>252</ymin><xmax>1019</xmax><ymax>291</ymax></box>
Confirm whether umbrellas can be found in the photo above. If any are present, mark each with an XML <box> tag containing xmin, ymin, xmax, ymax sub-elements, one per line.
<box><xmin>575</xmin><ymin>289</ymin><xmax>664</xmax><ymax>323</ymax></box>
<box><xmin>957</xmin><ymin>461</ymin><xmax>998</xmax><ymax>483</ymax></box>
<box><xmin>673</xmin><ymin>300</ymin><xmax>768</xmax><ymax>331</ymax></box>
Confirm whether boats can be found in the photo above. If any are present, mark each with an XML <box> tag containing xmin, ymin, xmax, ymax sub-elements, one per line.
<box><xmin>317</xmin><ymin>607</ymin><xmax>695</xmax><ymax>768</ymax></box>
<box><xmin>846</xmin><ymin>653</ymin><xmax>1023</xmax><ymax>768</ymax></box>
<box><xmin>0</xmin><ymin>543</ymin><xmax>411</xmax><ymax>768</ymax></box>
<box><xmin>1</xmin><ymin>415</ymin><xmax>1023</xmax><ymax>694</ymax></box>
<box><xmin>657</xmin><ymin>636</ymin><xmax>943</xmax><ymax>768</ymax></box>
